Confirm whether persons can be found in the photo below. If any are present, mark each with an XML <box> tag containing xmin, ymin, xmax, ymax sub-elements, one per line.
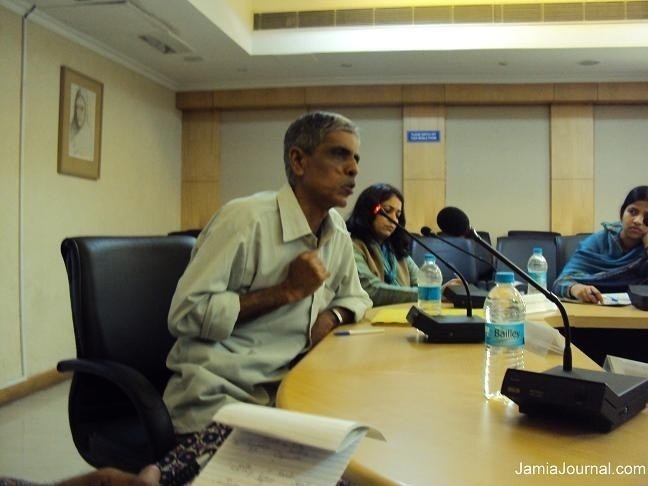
<box><xmin>346</xmin><ymin>182</ymin><xmax>469</xmax><ymax>309</ymax></box>
<box><xmin>0</xmin><ymin>463</ymin><xmax>161</xmax><ymax>485</ymax></box>
<box><xmin>162</xmin><ymin>110</ymin><xmax>374</xmax><ymax>448</ymax></box>
<box><xmin>550</xmin><ymin>185</ymin><xmax>648</xmax><ymax>306</ymax></box>
<box><xmin>67</xmin><ymin>86</ymin><xmax>94</xmax><ymax>161</ymax></box>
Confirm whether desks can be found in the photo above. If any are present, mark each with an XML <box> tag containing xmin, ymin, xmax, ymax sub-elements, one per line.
<box><xmin>276</xmin><ymin>300</ymin><xmax>648</xmax><ymax>485</ymax></box>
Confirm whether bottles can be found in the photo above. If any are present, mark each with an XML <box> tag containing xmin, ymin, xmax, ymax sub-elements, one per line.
<box><xmin>416</xmin><ymin>254</ymin><xmax>443</xmax><ymax>317</ymax></box>
<box><xmin>483</xmin><ymin>271</ymin><xmax>527</xmax><ymax>402</ymax></box>
<box><xmin>526</xmin><ymin>247</ymin><xmax>549</xmax><ymax>293</ymax></box>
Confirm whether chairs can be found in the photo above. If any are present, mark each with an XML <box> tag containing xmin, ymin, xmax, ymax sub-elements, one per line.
<box><xmin>57</xmin><ymin>231</ymin><xmax>598</xmax><ymax>475</ymax></box>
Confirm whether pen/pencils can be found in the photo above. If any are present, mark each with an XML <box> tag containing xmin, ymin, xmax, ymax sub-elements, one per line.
<box><xmin>333</xmin><ymin>329</ymin><xmax>385</xmax><ymax>335</ymax></box>
<box><xmin>453</xmin><ymin>272</ymin><xmax>460</xmax><ymax>279</ymax></box>
<box><xmin>607</xmin><ymin>295</ymin><xmax>618</xmax><ymax>301</ymax></box>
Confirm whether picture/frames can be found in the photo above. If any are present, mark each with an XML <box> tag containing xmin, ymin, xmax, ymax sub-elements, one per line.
<box><xmin>57</xmin><ymin>65</ymin><xmax>104</xmax><ymax>179</ymax></box>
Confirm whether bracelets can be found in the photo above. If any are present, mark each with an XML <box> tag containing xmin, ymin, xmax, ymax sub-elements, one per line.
<box><xmin>327</xmin><ymin>307</ymin><xmax>345</xmax><ymax>324</ymax></box>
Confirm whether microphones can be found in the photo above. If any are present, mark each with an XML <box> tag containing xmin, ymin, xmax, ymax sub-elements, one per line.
<box><xmin>436</xmin><ymin>206</ymin><xmax>648</xmax><ymax>432</ymax></box>
<box><xmin>626</xmin><ymin>212</ymin><xmax>648</xmax><ymax>312</ymax></box>
<box><xmin>421</xmin><ymin>227</ymin><xmax>497</xmax><ymax>308</ymax></box>
<box><xmin>361</xmin><ymin>193</ymin><xmax>487</xmax><ymax>344</ymax></box>
